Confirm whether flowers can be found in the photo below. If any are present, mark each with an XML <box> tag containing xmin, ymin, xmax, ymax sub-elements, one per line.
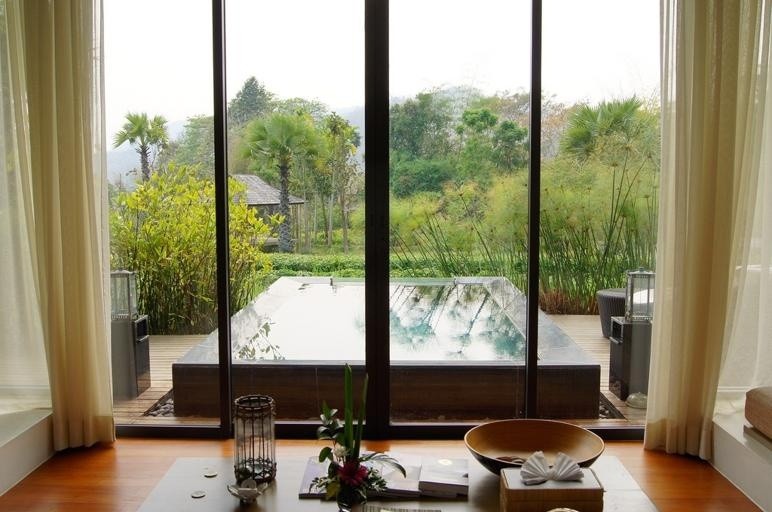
<box><xmin>312</xmin><ymin>362</ymin><xmax>405</xmax><ymax>501</ymax></box>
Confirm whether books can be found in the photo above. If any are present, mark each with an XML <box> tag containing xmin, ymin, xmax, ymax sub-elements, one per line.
<box><xmin>298</xmin><ymin>451</ymin><xmax>469</xmax><ymax>500</ymax></box>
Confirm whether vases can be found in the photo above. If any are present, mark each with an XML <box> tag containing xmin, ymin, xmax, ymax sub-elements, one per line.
<box><xmin>336</xmin><ymin>496</ymin><xmax>365</xmax><ymax>511</ymax></box>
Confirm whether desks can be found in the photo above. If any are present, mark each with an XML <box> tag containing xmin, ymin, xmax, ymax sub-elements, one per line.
<box><xmin>135</xmin><ymin>455</ymin><xmax>659</xmax><ymax>512</ymax></box>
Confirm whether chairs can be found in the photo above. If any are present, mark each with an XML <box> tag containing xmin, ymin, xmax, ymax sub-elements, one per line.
<box><xmin>596</xmin><ymin>287</ymin><xmax>654</xmax><ymax>341</ymax></box>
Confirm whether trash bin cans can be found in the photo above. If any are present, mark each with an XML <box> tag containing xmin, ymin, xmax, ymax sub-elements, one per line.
<box><xmin>596</xmin><ymin>288</ymin><xmax>627</xmax><ymax>339</ymax></box>
